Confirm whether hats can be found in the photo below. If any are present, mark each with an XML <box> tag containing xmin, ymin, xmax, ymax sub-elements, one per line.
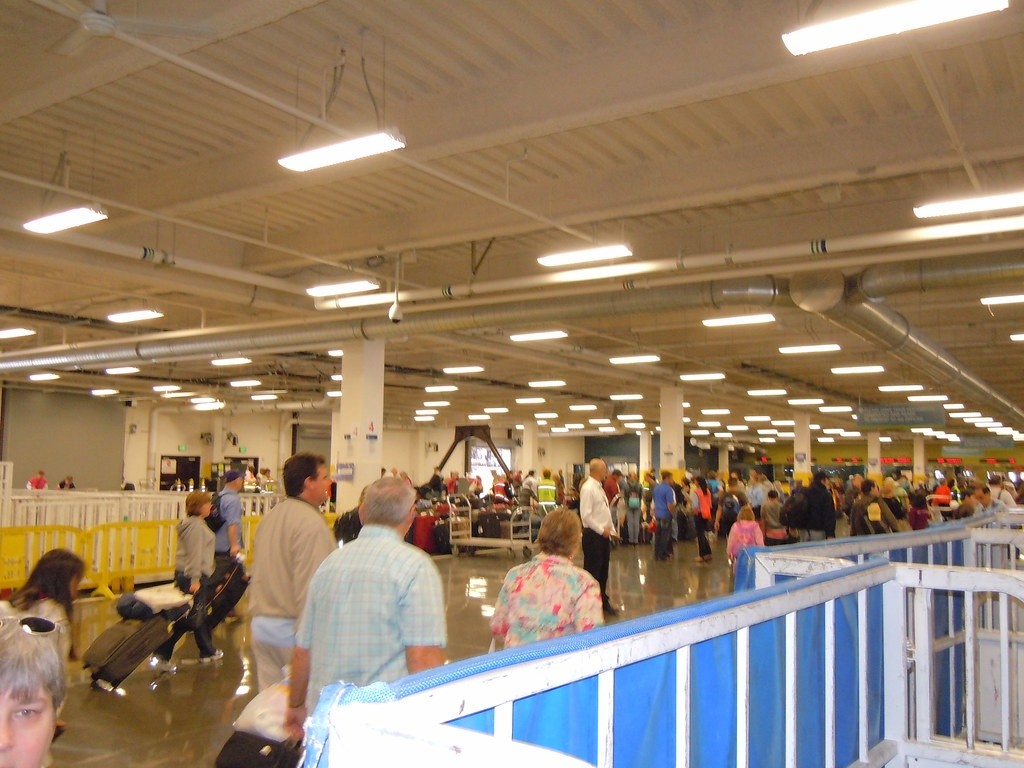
<box><xmin>219</xmin><ymin>471</ymin><xmax>248</xmax><ymax>484</ymax></box>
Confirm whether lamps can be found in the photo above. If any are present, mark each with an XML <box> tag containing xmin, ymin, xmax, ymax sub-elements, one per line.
<box><xmin>0</xmin><ymin>274</ymin><xmax>38</xmax><ymax>340</ymax></box>
<box><xmin>277</xmin><ymin>35</ymin><xmax>409</xmax><ymax>174</ymax></box>
<box><xmin>305</xmin><ymin>256</ymin><xmax>384</xmax><ymax>299</ymax></box>
<box><xmin>105</xmin><ymin>270</ymin><xmax>166</xmax><ymax>324</ymax></box>
<box><xmin>537</xmin><ymin>218</ymin><xmax>634</xmax><ymax>269</ymax></box>
<box><xmin>23</xmin><ymin>129</ymin><xmax>109</xmax><ymax>232</ymax></box>
<box><xmin>780</xmin><ymin>0</ymin><xmax>1010</xmax><ymax>56</ymax></box>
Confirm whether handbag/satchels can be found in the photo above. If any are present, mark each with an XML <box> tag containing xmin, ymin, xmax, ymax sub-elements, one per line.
<box><xmin>134</xmin><ymin>582</ymin><xmax>195</xmax><ymax>614</ymax></box>
<box><xmin>648</xmin><ymin>519</ymin><xmax>657</xmax><ymax>533</ymax></box>
<box><xmin>232</xmin><ymin>664</ymin><xmax>308</xmax><ymax>742</ymax></box>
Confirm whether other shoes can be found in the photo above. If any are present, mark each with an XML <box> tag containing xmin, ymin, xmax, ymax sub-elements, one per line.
<box><xmin>198</xmin><ymin>649</ymin><xmax>224</xmax><ymax>664</ymax></box>
<box><xmin>603</xmin><ymin>602</ymin><xmax>619</xmax><ymax>616</ymax></box>
<box><xmin>150</xmin><ymin>652</ymin><xmax>177</xmax><ymax>672</ymax></box>
<box><xmin>692</xmin><ymin>554</ymin><xmax>713</xmax><ymax>562</ymax></box>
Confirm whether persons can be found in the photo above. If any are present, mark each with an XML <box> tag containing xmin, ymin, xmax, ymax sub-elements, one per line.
<box><xmin>381</xmin><ymin>467</ymin><xmax>411</xmax><ymax>485</ymax></box>
<box><xmin>214</xmin><ymin>471</ymin><xmax>246</xmax><ymax>622</ymax></box>
<box><xmin>150</xmin><ymin>491</ymin><xmax>224</xmax><ymax>671</ymax></box>
<box><xmin>435</xmin><ymin>467</ymin><xmax>483</xmax><ymax>498</ymax></box>
<box><xmin>0</xmin><ymin>618</ymin><xmax>66</xmax><ymax>768</ymax></box>
<box><xmin>246</xmin><ymin>466</ymin><xmax>277</xmax><ymax>511</ymax></box>
<box><xmin>58</xmin><ymin>475</ymin><xmax>75</xmax><ymax>490</ymax></box>
<box><xmin>788</xmin><ymin>470</ymin><xmax>1024</xmax><ymax>559</ymax></box>
<box><xmin>333</xmin><ymin>485</ymin><xmax>370</xmax><ymax>546</ymax></box>
<box><xmin>121</xmin><ymin>477</ymin><xmax>135</xmax><ymax>490</ymax></box>
<box><xmin>285</xmin><ymin>476</ymin><xmax>447</xmax><ymax>738</ymax></box>
<box><xmin>580</xmin><ymin>458</ymin><xmax>619</xmax><ymax>613</ymax></box>
<box><xmin>489</xmin><ymin>510</ymin><xmax>605</xmax><ymax>650</ymax></box>
<box><xmin>26</xmin><ymin>471</ymin><xmax>48</xmax><ymax>499</ymax></box>
<box><xmin>490</xmin><ymin>468</ymin><xmax>567</xmax><ymax>531</ymax></box>
<box><xmin>11</xmin><ymin>547</ymin><xmax>87</xmax><ymax>737</ymax></box>
<box><xmin>602</xmin><ymin>467</ymin><xmax>788</xmax><ymax>571</ymax></box>
<box><xmin>250</xmin><ymin>453</ymin><xmax>337</xmax><ymax>691</ymax></box>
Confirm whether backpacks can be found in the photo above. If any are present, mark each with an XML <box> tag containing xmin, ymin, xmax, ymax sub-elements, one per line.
<box><xmin>723</xmin><ymin>490</ymin><xmax>740</xmax><ymax>519</ymax></box>
<box><xmin>204</xmin><ymin>491</ymin><xmax>233</xmax><ymax>533</ymax></box>
<box><xmin>731</xmin><ymin>520</ymin><xmax>758</xmax><ymax>558</ymax></box>
<box><xmin>778</xmin><ymin>487</ymin><xmax>827</xmax><ymax>530</ymax></box>
<box><xmin>856</xmin><ymin>496</ymin><xmax>889</xmax><ymax>535</ymax></box>
<box><xmin>627</xmin><ymin>480</ymin><xmax>641</xmax><ymax>510</ymax></box>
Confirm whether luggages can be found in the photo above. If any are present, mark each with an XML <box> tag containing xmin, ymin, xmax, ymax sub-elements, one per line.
<box><xmin>81</xmin><ymin>590</ymin><xmax>197</xmax><ymax>691</ymax></box>
<box><xmin>205</xmin><ymin>552</ymin><xmax>252</xmax><ymax>630</ymax></box>
<box><xmin>405</xmin><ymin>493</ymin><xmax>523</xmax><ymax>555</ymax></box>
<box><xmin>213</xmin><ymin>731</ymin><xmax>303</xmax><ymax>768</ymax></box>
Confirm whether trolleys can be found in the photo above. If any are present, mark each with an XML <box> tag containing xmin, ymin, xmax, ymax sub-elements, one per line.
<box><xmin>446</xmin><ymin>490</ymin><xmax>533</xmax><ymax>560</ymax></box>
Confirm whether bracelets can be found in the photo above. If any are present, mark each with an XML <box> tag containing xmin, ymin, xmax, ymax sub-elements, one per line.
<box><xmin>289</xmin><ymin>700</ymin><xmax>304</xmax><ymax>708</ymax></box>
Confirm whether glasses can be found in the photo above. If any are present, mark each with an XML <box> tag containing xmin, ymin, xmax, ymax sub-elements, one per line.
<box><xmin>0</xmin><ymin>615</ymin><xmax>61</xmax><ymax>635</ymax></box>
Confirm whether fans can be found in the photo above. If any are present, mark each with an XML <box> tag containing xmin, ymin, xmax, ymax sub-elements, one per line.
<box><xmin>30</xmin><ymin>0</ymin><xmax>218</xmax><ymax>58</ymax></box>
